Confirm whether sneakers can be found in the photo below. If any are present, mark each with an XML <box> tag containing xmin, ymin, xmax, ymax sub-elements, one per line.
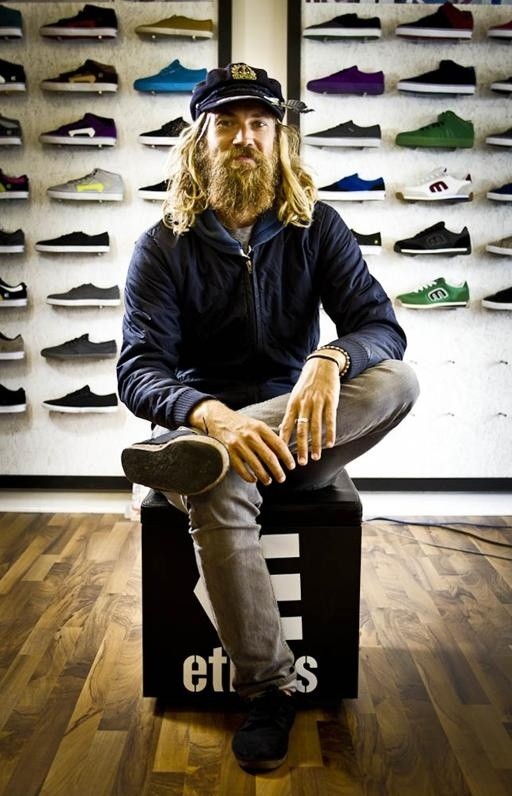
<box><xmin>39</xmin><ymin>5</ymin><xmax>117</xmax><ymax>39</ymax></box>
<box><xmin>303</xmin><ymin>119</ymin><xmax>384</xmax><ymax>150</ymax></box>
<box><xmin>307</xmin><ymin>65</ymin><xmax>386</xmax><ymax>97</ymax></box>
<box><xmin>320</xmin><ymin>175</ymin><xmax>389</xmax><ymax>204</ymax></box>
<box><xmin>46</xmin><ymin>169</ymin><xmax>123</xmax><ymax>202</ymax></box>
<box><xmin>394</xmin><ymin>58</ymin><xmax>478</xmax><ymax>100</ymax></box>
<box><xmin>0</xmin><ymin>384</ymin><xmax>27</xmax><ymax>413</ymax></box>
<box><xmin>1</xmin><ymin>58</ymin><xmax>26</xmax><ymax>92</ymax></box>
<box><xmin>1</xmin><ymin>4</ymin><xmax>23</xmax><ymax>39</ymax></box>
<box><xmin>135</xmin><ymin>12</ymin><xmax>215</xmax><ymax>204</ymax></box>
<box><xmin>349</xmin><ymin>219</ymin><xmax>472</xmax><ymax>313</ymax></box>
<box><xmin>1</xmin><ymin>333</ymin><xmax>25</xmax><ymax>361</ymax></box>
<box><xmin>302</xmin><ymin>12</ymin><xmax>385</xmax><ymax>42</ymax></box>
<box><xmin>0</xmin><ymin>228</ymin><xmax>26</xmax><ymax>253</ymax></box>
<box><xmin>121</xmin><ymin>424</ymin><xmax>229</xmax><ymax>499</ymax></box>
<box><xmin>226</xmin><ymin>685</ymin><xmax>301</xmax><ymax>776</ymax></box>
<box><xmin>482</xmin><ymin>19</ymin><xmax>512</xmax><ymax>312</ymax></box>
<box><xmin>1</xmin><ymin>114</ymin><xmax>22</xmax><ymax>146</ymax></box>
<box><xmin>41</xmin><ymin>386</ymin><xmax>119</xmax><ymax>414</ymax></box>
<box><xmin>0</xmin><ymin>169</ymin><xmax>29</xmax><ymax>199</ymax></box>
<box><xmin>35</xmin><ymin>232</ymin><xmax>110</xmax><ymax>253</ymax></box>
<box><xmin>45</xmin><ymin>283</ymin><xmax>119</xmax><ymax>307</ymax></box>
<box><xmin>39</xmin><ymin>58</ymin><xmax>118</xmax><ymax>93</ymax></box>
<box><xmin>41</xmin><ymin>333</ymin><xmax>116</xmax><ymax>358</ymax></box>
<box><xmin>39</xmin><ymin>113</ymin><xmax>116</xmax><ymax>146</ymax></box>
<box><xmin>395</xmin><ymin>113</ymin><xmax>476</xmax><ymax>149</ymax></box>
<box><xmin>394</xmin><ymin>1</ymin><xmax>476</xmax><ymax>39</ymax></box>
<box><xmin>396</xmin><ymin>167</ymin><xmax>475</xmax><ymax>204</ymax></box>
<box><xmin>1</xmin><ymin>279</ymin><xmax>29</xmax><ymax>307</ymax></box>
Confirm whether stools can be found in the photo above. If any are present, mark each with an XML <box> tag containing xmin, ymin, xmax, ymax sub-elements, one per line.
<box><xmin>141</xmin><ymin>468</ymin><xmax>361</xmax><ymax>702</ymax></box>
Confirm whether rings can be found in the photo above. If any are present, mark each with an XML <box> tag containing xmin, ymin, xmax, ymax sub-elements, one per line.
<box><xmin>296</xmin><ymin>417</ymin><xmax>309</xmax><ymax>424</ymax></box>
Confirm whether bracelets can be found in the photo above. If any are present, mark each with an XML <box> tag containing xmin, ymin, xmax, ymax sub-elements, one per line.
<box><xmin>304</xmin><ymin>356</ymin><xmax>339</xmax><ymax>367</ymax></box>
<box><xmin>314</xmin><ymin>346</ymin><xmax>352</xmax><ymax>377</ymax></box>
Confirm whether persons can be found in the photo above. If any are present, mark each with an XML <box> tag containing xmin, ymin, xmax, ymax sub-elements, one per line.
<box><xmin>113</xmin><ymin>61</ymin><xmax>417</xmax><ymax>774</ymax></box>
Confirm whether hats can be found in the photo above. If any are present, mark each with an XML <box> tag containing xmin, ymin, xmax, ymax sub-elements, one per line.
<box><xmin>188</xmin><ymin>60</ymin><xmax>284</xmax><ymax>121</ymax></box>
